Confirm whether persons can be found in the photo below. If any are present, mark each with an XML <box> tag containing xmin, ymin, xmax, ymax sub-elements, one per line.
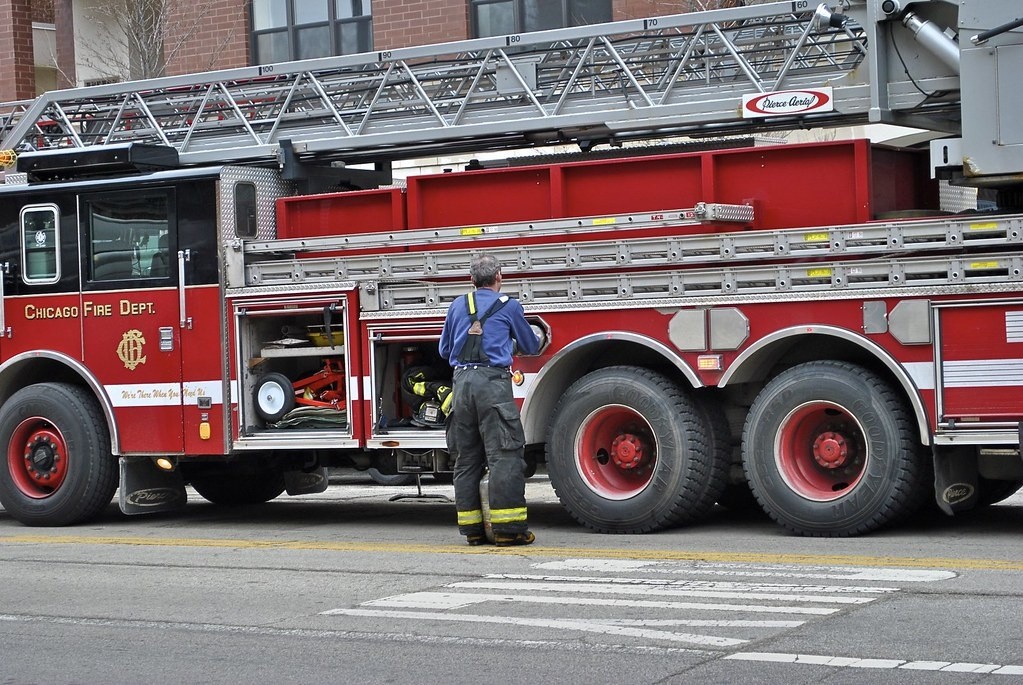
<box><xmin>439</xmin><ymin>256</ymin><xmax>544</xmax><ymax>547</ymax></box>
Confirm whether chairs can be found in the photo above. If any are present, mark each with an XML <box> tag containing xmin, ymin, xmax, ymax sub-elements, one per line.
<box><xmin>94</xmin><ymin>223</ymin><xmax>133</xmax><ymax>281</ymax></box>
<box><xmin>150</xmin><ymin>234</ymin><xmax>168</xmax><ymax>277</ymax></box>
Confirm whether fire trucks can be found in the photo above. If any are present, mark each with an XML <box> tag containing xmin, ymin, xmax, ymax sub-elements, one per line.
<box><xmin>1</xmin><ymin>0</ymin><xmax>1023</xmax><ymax>529</ymax></box>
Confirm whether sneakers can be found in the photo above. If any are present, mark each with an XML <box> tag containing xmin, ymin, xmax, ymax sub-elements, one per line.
<box><xmin>467</xmin><ymin>519</ymin><xmax>495</xmax><ymax>546</ymax></box>
<box><xmin>495</xmin><ymin>528</ymin><xmax>535</xmax><ymax>546</ymax></box>
